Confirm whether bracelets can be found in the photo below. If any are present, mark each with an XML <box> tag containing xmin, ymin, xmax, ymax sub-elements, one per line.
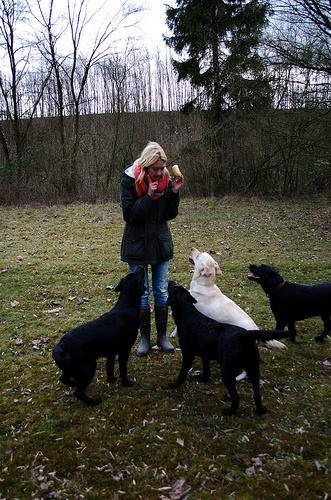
<box><xmin>148</xmin><ymin>193</ymin><xmax>151</xmax><ymax>196</ymax></box>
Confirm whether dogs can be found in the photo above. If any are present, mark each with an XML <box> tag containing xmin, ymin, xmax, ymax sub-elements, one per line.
<box><xmin>247</xmin><ymin>263</ymin><xmax>331</xmax><ymax>343</ymax></box>
<box><xmin>166</xmin><ymin>280</ymin><xmax>291</xmax><ymax>416</ymax></box>
<box><xmin>169</xmin><ymin>246</ymin><xmax>288</xmax><ymax>382</ymax></box>
<box><xmin>52</xmin><ymin>267</ymin><xmax>148</xmax><ymax>406</ymax></box>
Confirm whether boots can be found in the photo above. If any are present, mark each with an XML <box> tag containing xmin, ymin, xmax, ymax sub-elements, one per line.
<box><xmin>154</xmin><ymin>307</ymin><xmax>175</xmax><ymax>351</ymax></box>
<box><xmin>137</xmin><ymin>308</ymin><xmax>151</xmax><ymax>356</ymax></box>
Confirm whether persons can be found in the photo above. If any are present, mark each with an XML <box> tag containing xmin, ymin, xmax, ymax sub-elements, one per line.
<box><xmin>120</xmin><ymin>142</ymin><xmax>184</xmax><ymax>356</ymax></box>
